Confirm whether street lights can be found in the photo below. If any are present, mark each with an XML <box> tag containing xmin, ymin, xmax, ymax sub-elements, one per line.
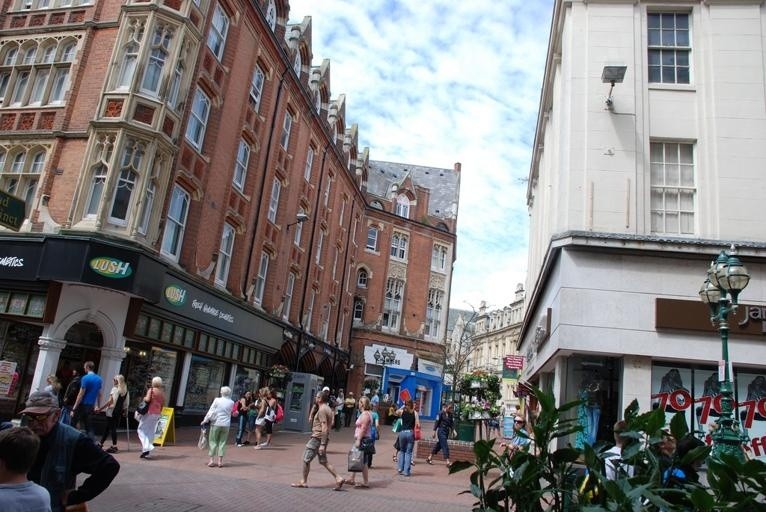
<box><xmin>699</xmin><ymin>244</ymin><xmax>751</xmax><ymax>480</ymax></box>
<box><xmin>373</xmin><ymin>347</ymin><xmax>395</xmax><ymax>406</ymax></box>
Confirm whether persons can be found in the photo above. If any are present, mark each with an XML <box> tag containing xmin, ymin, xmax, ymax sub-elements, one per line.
<box><xmin>134</xmin><ymin>376</ymin><xmax>164</xmax><ymax>458</ymax></box>
<box><xmin>96</xmin><ymin>374</ymin><xmax>130</xmax><ymax>453</ymax></box>
<box><xmin>0</xmin><ymin>426</ymin><xmax>53</xmax><ymax>512</ymax></box>
<box><xmin>291</xmin><ymin>386</ymin><xmax>420</xmax><ymax>491</ymax></box>
<box><xmin>70</xmin><ymin>360</ymin><xmax>104</xmax><ymax>435</ymax></box>
<box><xmin>18</xmin><ymin>389</ymin><xmax>120</xmax><ymax>512</ymax></box>
<box><xmin>512</xmin><ymin>416</ymin><xmax>529</xmax><ymax>447</ymax></box>
<box><xmin>45</xmin><ymin>374</ymin><xmax>62</xmax><ymax>406</ymax></box>
<box><xmin>236</xmin><ymin>387</ymin><xmax>277</xmax><ymax>450</ymax></box>
<box><xmin>201</xmin><ymin>386</ymin><xmax>235</xmax><ymax>467</ymax></box>
<box><xmin>59</xmin><ymin>367</ymin><xmax>82</xmax><ymax>427</ymax></box>
<box><xmin>426</xmin><ymin>404</ymin><xmax>458</xmax><ymax>466</ymax></box>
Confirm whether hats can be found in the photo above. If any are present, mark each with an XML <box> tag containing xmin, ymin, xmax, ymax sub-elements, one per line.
<box><xmin>18</xmin><ymin>392</ymin><xmax>59</xmax><ymax>415</ymax></box>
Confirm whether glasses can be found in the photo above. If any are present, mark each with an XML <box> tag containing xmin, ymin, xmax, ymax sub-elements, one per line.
<box><xmin>24</xmin><ymin>415</ymin><xmax>49</xmax><ymax>422</ymax></box>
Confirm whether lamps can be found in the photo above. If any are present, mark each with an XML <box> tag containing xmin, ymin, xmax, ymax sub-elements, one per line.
<box><xmin>287</xmin><ymin>213</ymin><xmax>309</xmax><ymax>229</ymax></box>
<box><xmin>599</xmin><ymin>66</ymin><xmax>628</xmax><ymax>106</ymax></box>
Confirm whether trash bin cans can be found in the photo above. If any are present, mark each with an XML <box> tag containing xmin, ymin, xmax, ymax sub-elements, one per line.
<box><xmin>458</xmin><ymin>423</ymin><xmax>474</xmax><ymax>440</ymax></box>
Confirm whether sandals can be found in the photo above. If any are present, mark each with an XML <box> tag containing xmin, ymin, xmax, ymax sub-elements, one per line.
<box><xmin>426</xmin><ymin>457</ymin><xmax>434</xmax><ymax>465</ymax></box>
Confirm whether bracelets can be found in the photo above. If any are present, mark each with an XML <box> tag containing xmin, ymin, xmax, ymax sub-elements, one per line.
<box><xmin>72</xmin><ymin>409</ymin><xmax>76</xmax><ymax>412</ymax></box>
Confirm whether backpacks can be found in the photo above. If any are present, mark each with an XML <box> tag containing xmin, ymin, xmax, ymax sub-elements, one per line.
<box><xmin>231</xmin><ymin>400</ymin><xmax>240</xmax><ymax>417</ymax></box>
<box><xmin>265</xmin><ymin>399</ymin><xmax>276</xmax><ymax>423</ymax></box>
<box><xmin>274</xmin><ymin>402</ymin><xmax>284</xmax><ymax>423</ymax></box>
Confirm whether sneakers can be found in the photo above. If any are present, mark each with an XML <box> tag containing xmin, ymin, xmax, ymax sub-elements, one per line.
<box><xmin>106</xmin><ymin>446</ymin><xmax>117</xmax><ymax>453</ymax></box>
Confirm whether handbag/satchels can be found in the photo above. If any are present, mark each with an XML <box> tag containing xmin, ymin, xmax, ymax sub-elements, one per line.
<box><xmin>392</xmin><ymin>418</ymin><xmax>403</xmax><ymax>432</ymax></box>
<box><xmin>348</xmin><ymin>451</ymin><xmax>364</xmax><ymax>472</ymax></box>
<box><xmin>137</xmin><ymin>400</ymin><xmax>150</xmax><ymax>415</ymax></box>
<box><xmin>414</xmin><ymin>424</ymin><xmax>422</xmax><ymax>440</ymax></box>
<box><xmin>198</xmin><ymin>426</ymin><xmax>208</xmax><ymax>451</ymax></box>
